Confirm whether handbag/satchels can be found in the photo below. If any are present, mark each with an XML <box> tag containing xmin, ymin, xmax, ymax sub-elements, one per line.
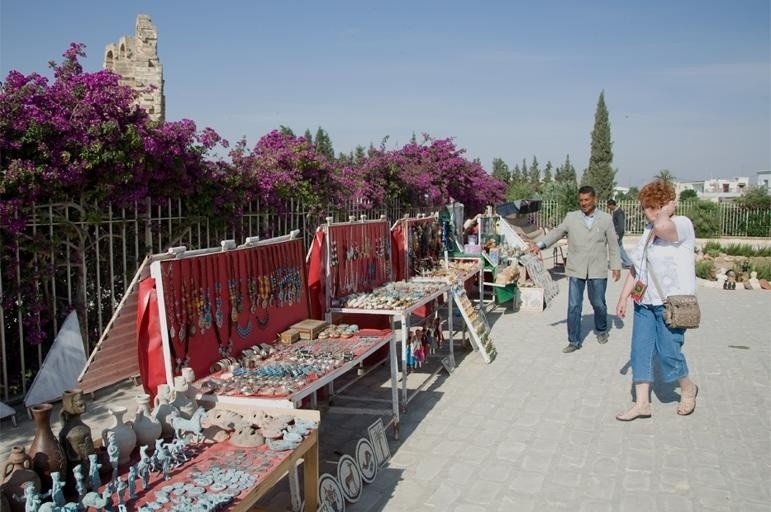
<box><xmin>662</xmin><ymin>293</ymin><xmax>701</xmax><ymax>329</ymax></box>
<box><xmin>629</xmin><ymin>279</ymin><xmax>649</xmax><ymax>303</ymax></box>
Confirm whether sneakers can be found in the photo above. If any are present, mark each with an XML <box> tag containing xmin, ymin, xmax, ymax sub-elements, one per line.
<box><xmin>596</xmin><ymin>329</ymin><xmax>609</xmax><ymax>345</ymax></box>
<box><xmin>562</xmin><ymin>343</ymin><xmax>582</xmax><ymax>353</ymax></box>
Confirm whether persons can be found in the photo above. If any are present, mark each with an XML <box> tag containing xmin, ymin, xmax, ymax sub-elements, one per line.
<box><xmin>407</xmin><ymin>317</ymin><xmax>444</xmax><ymax>372</ymax></box>
<box><xmin>605</xmin><ymin>197</ymin><xmax>635</xmax><ymax>272</ymax></box>
<box><xmin>527</xmin><ymin>184</ymin><xmax>623</xmax><ymax>354</ymax></box>
<box><xmin>9</xmin><ymin>431</ymin><xmax>206</xmax><ymax>512</ymax></box>
<box><xmin>614</xmin><ymin>177</ymin><xmax>700</xmax><ymax>421</ymax></box>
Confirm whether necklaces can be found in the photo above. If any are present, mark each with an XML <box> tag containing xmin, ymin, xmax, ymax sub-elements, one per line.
<box><xmin>326</xmin><ymin>220</ymin><xmax>391</xmax><ymax>301</ymax></box>
<box><xmin>159</xmin><ymin>239</ymin><xmax>306</xmax><ymax>376</ymax></box>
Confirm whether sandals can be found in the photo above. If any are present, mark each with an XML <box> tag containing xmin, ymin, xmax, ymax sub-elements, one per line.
<box><xmin>615</xmin><ymin>401</ymin><xmax>656</xmax><ymax>421</ymax></box>
<box><xmin>676</xmin><ymin>383</ymin><xmax>699</xmax><ymax>415</ymax></box>
